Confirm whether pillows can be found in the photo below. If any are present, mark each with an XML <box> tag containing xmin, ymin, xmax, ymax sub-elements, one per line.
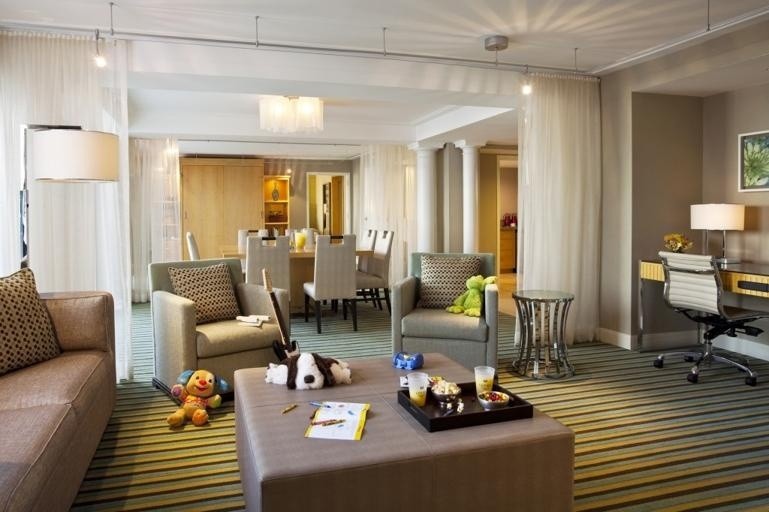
<box><xmin>418</xmin><ymin>255</ymin><xmax>481</xmax><ymax>308</ymax></box>
<box><xmin>168</xmin><ymin>262</ymin><xmax>241</xmax><ymax>325</ymax></box>
<box><xmin>0</xmin><ymin>267</ymin><xmax>60</xmax><ymax>378</ymax></box>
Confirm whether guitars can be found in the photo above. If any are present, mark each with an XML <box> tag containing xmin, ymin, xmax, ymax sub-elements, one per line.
<box><xmin>262</xmin><ymin>268</ymin><xmax>300</xmax><ymax>361</ymax></box>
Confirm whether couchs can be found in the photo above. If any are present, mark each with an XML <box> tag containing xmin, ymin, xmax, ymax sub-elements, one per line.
<box><xmin>391</xmin><ymin>252</ymin><xmax>498</xmax><ymax>375</ymax></box>
<box><xmin>0</xmin><ymin>289</ymin><xmax>117</xmax><ymax>511</ymax></box>
<box><xmin>148</xmin><ymin>258</ymin><xmax>290</xmax><ymax>405</ymax></box>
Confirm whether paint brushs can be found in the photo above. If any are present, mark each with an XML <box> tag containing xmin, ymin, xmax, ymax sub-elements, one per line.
<box><xmin>322</xmin><ymin>419</ymin><xmax>345</xmax><ymax>426</ymax></box>
<box><xmin>309</xmin><ymin>402</ymin><xmax>331</xmax><ymax>409</ymax></box>
<box><xmin>309</xmin><ymin>409</ymin><xmax>317</xmax><ymax>419</ymax></box>
<box><xmin>311</xmin><ymin>420</ymin><xmax>334</xmax><ymax>425</ymax></box>
<box><xmin>282</xmin><ymin>404</ymin><xmax>297</xmax><ymax>415</ymax></box>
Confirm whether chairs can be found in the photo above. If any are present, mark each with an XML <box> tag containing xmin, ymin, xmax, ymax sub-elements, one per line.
<box><xmin>350</xmin><ymin>230</ymin><xmax>395</xmax><ymax>315</ymax></box>
<box><xmin>186</xmin><ymin>231</ymin><xmax>201</xmax><ymax>260</ymax></box>
<box><xmin>653</xmin><ymin>249</ymin><xmax>769</xmax><ymax>386</ymax></box>
<box><xmin>245</xmin><ymin>235</ymin><xmax>290</xmax><ymax>302</ymax></box>
<box><xmin>238</xmin><ymin>229</ymin><xmax>268</xmax><ymax>274</ymax></box>
<box><xmin>355</xmin><ymin>228</ymin><xmax>377</xmax><ymax>277</ymax></box>
<box><xmin>304</xmin><ymin>234</ymin><xmax>358</xmax><ymax>334</ymax></box>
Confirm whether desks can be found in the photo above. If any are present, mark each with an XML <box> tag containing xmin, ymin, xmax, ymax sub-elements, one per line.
<box><xmin>635</xmin><ymin>257</ymin><xmax>769</xmax><ymax>351</ymax></box>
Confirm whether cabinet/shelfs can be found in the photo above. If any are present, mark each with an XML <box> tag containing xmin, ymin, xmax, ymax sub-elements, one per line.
<box><xmin>178</xmin><ymin>158</ymin><xmax>289</xmax><ymax>256</ymax></box>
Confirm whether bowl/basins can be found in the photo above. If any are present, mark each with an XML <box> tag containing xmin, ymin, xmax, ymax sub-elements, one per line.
<box><xmin>477</xmin><ymin>391</ymin><xmax>509</xmax><ymax>411</ymax></box>
<box><xmin>431</xmin><ymin>386</ymin><xmax>461</xmax><ymax>404</ymax></box>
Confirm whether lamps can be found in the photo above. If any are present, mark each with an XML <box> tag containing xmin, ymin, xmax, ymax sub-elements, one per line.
<box><xmin>34</xmin><ymin>130</ymin><xmax>120</xmax><ymax>182</ymax></box>
<box><xmin>522</xmin><ymin>71</ymin><xmax>532</xmax><ymax>95</ymax></box>
<box><xmin>260</xmin><ymin>96</ymin><xmax>325</xmax><ymax>130</ymax></box>
<box><xmin>690</xmin><ymin>204</ymin><xmax>746</xmax><ymax>263</ymax></box>
<box><xmin>94</xmin><ymin>36</ymin><xmax>105</xmax><ymax>68</ymax></box>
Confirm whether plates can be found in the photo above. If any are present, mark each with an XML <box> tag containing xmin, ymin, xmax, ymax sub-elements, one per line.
<box><xmin>397</xmin><ymin>382</ymin><xmax>533</xmax><ymax>434</ymax></box>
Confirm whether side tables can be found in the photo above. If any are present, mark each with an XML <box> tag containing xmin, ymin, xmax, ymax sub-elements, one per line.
<box><xmin>511</xmin><ymin>289</ymin><xmax>575</xmax><ymax>380</ymax></box>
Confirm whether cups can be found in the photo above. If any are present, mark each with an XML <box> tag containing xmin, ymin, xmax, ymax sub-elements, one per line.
<box><xmin>475</xmin><ymin>366</ymin><xmax>494</xmax><ymax>395</ymax></box>
<box><xmin>296</xmin><ymin>232</ymin><xmax>306</xmax><ymax>252</ymax></box>
<box><xmin>407</xmin><ymin>371</ymin><xmax>428</xmax><ymax>407</ymax></box>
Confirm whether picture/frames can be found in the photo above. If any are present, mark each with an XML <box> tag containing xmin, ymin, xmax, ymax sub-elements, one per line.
<box><xmin>736</xmin><ymin>130</ymin><xmax>769</xmax><ymax>194</ymax></box>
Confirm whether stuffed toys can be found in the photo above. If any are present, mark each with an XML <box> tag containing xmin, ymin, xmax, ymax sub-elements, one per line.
<box><xmin>446</xmin><ymin>275</ymin><xmax>496</xmax><ymax>317</ymax></box>
<box><xmin>167</xmin><ymin>370</ymin><xmax>228</xmax><ymax>427</ymax></box>
<box><xmin>264</xmin><ymin>353</ymin><xmax>351</xmax><ymax>390</ymax></box>
